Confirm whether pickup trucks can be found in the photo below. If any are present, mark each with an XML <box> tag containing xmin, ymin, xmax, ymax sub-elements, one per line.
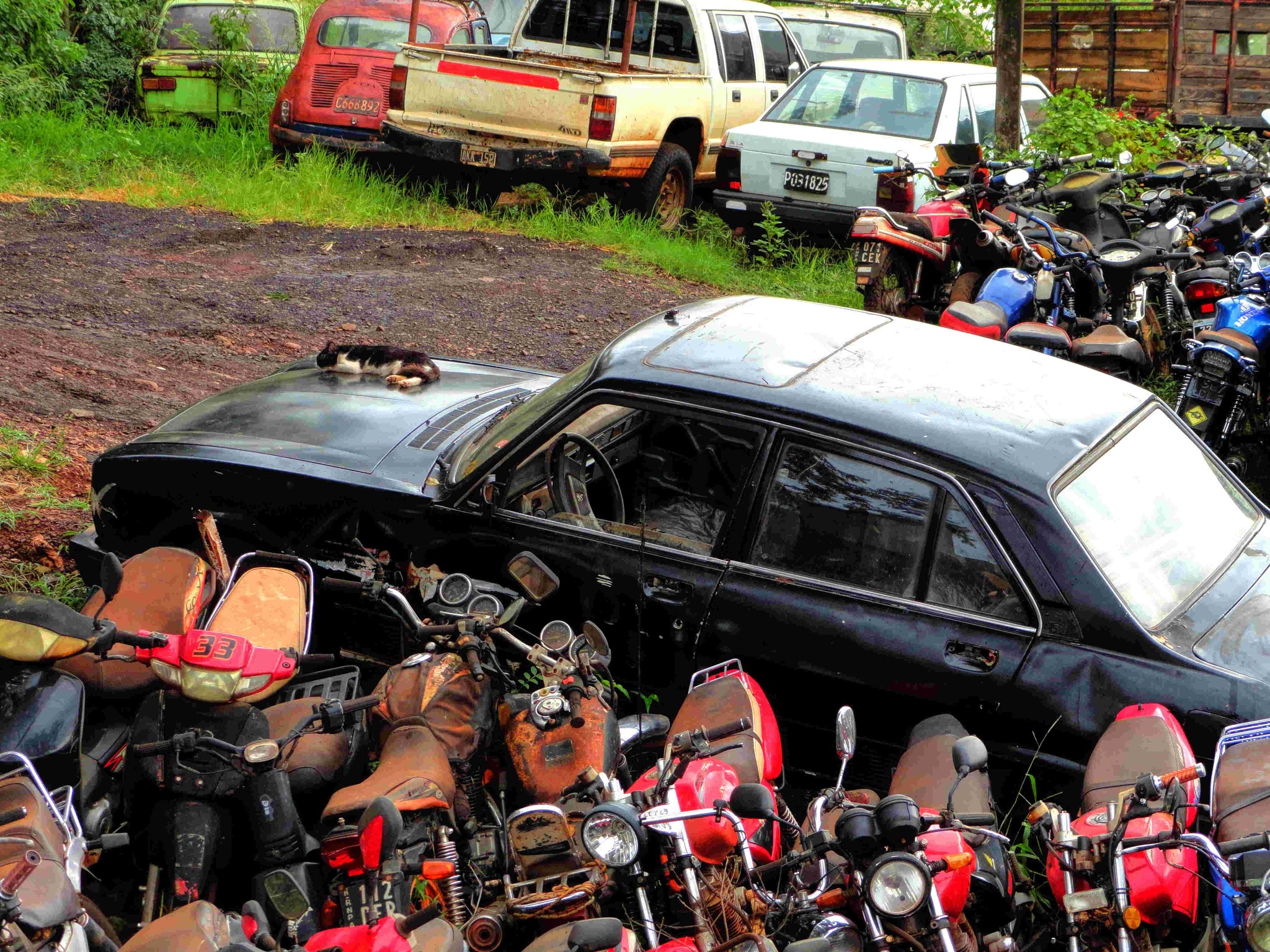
<box><xmin>378</xmin><ymin>0</ymin><xmax>810</xmax><ymax>237</ymax></box>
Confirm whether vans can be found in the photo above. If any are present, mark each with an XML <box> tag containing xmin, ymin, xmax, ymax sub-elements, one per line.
<box><xmin>768</xmin><ymin>4</ymin><xmax>912</xmax><ymax>102</ymax></box>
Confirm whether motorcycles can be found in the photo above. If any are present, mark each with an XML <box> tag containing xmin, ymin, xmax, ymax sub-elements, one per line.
<box><xmin>848</xmin><ymin>132</ymin><xmax>1270</xmax><ymax>490</ymax></box>
<box><xmin>0</xmin><ymin>508</ymin><xmax>1268</xmax><ymax>951</ymax></box>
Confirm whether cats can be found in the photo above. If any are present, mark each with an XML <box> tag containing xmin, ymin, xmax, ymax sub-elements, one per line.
<box><xmin>315</xmin><ymin>340</ymin><xmax>441</xmax><ymax>388</ymax></box>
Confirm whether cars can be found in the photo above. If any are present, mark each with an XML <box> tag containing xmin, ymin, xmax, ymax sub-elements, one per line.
<box><xmin>134</xmin><ymin>0</ymin><xmax>310</xmax><ymax>137</ymax></box>
<box><xmin>268</xmin><ymin>1</ymin><xmax>507</xmax><ymax>166</ymax></box>
<box><xmin>710</xmin><ymin>49</ymin><xmax>1061</xmax><ymax>268</ymax></box>
<box><xmin>92</xmin><ymin>282</ymin><xmax>1270</xmax><ymax>830</ymax></box>
